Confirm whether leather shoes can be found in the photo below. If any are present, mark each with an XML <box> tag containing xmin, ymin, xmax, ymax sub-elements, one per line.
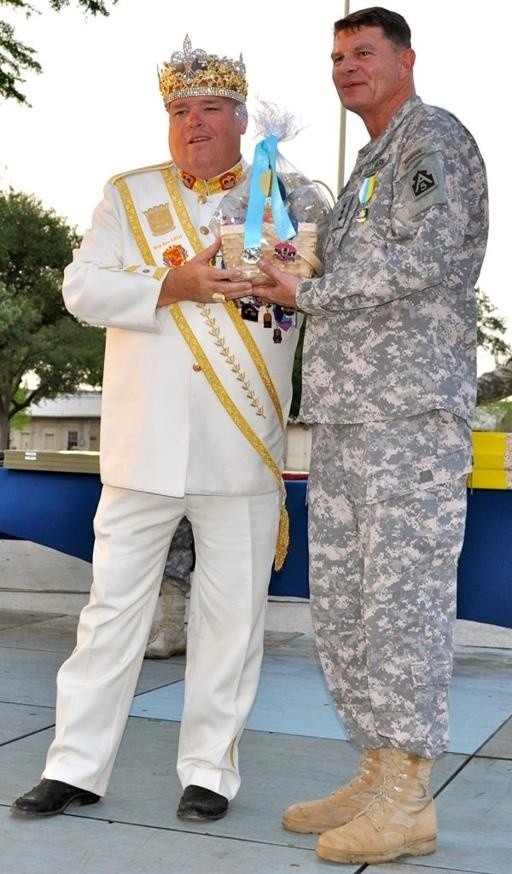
<box><xmin>10</xmin><ymin>776</ymin><xmax>101</xmax><ymax>820</ymax></box>
<box><xmin>177</xmin><ymin>785</ymin><xmax>228</xmax><ymax>824</ymax></box>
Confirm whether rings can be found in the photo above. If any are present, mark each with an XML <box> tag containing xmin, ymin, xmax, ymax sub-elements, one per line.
<box><xmin>212</xmin><ymin>292</ymin><xmax>227</xmax><ymax>305</ymax></box>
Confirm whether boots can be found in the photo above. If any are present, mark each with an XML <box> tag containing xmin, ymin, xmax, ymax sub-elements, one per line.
<box><xmin>282</xmin><ymin>744</ymin><xmax>439</xmax><ymax>864</ymax></box>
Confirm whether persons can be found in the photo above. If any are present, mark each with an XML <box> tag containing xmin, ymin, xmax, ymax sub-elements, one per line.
<box><xmin>7</xmin><ymin>27</ymin><xmax>326</xmax><ymax>825</ymax></box>
<box><xmin>234</xmin><ymin>4</ymin><xmax>497</xmax><ymax>871</ymax></box>
<box><xmin>141</xmin><ymin>516</ymin><xmax>197</xmax><ymax>660</ymax></box>
